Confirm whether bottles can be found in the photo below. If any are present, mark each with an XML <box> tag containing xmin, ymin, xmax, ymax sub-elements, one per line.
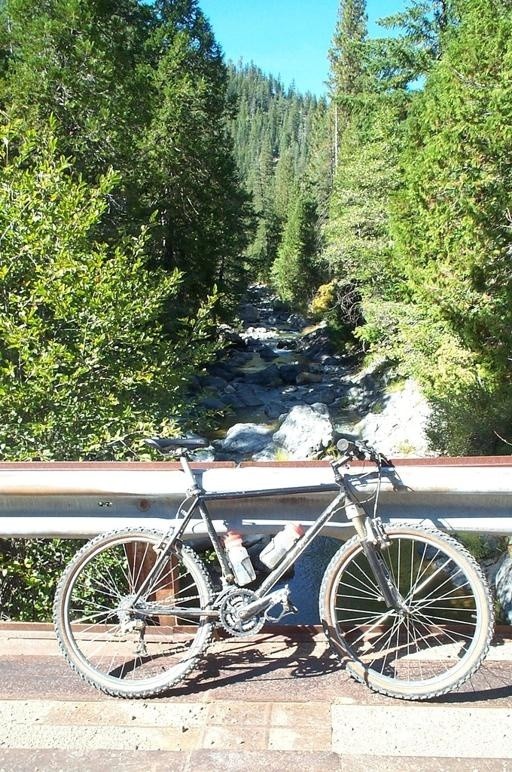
<box><xmin>223</xmin><ymin>528</ymin><xmax>258</xmax><ymax>589</ymax></box>
<box><xmin>258</xmin><ymin>520</ymin><xmax>305</xmax><ymax>569</ymax></box>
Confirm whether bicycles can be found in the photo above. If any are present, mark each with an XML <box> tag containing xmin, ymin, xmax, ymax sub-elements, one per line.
<box><xmin>49</xmin><ymin>435</ymin><xmax>496</xmax><ymax>705</ymax></box>
